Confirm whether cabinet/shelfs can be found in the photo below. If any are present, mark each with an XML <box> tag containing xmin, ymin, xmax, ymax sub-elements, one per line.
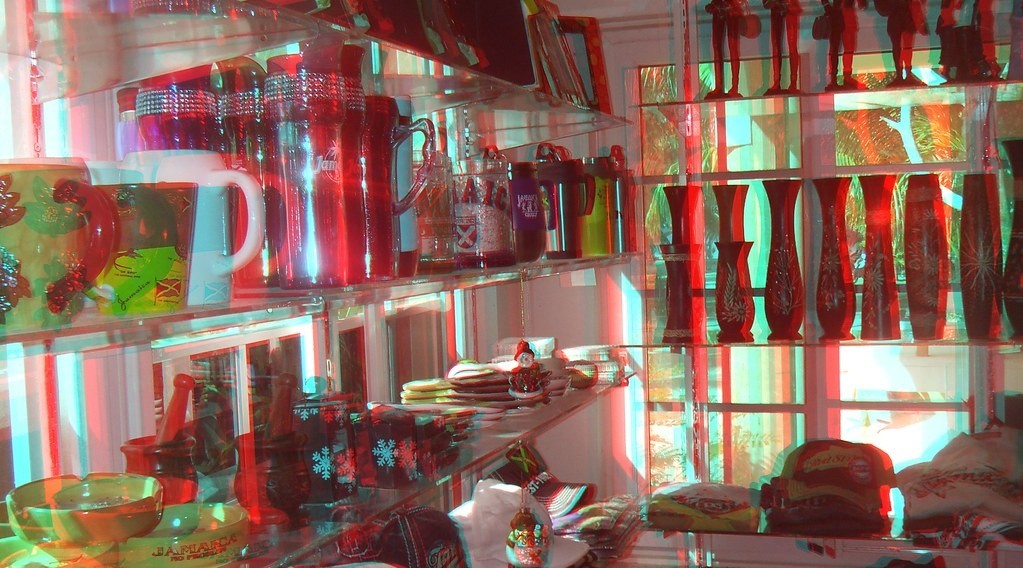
<box><xmin>647</xmin><ymin>78</ymin><xmax>1023</xmax><ymax>547</ymax></box>
<box><xmin>0</xmin><ymin>0</ymin><xmax>640</xmax><ymax>568</ymax></box>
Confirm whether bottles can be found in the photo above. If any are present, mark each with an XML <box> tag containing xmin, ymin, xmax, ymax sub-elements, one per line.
<box><xmin>658</xmin><ymin>138</ymin><xmax>1023</xmax><ymax>344</ymax></box>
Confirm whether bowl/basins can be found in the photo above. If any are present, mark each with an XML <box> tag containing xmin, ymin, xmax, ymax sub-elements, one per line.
<box><xmin>4</xmin><ymin>471</ymin><xmax>164</xmax><ymax>560</ymax></box>
<box><xmin>128</xmin><ymin>503</ymin><xmax>247</xmax><ymax>567</ymax></box>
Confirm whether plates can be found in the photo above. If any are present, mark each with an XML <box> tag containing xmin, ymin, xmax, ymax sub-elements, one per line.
<box><xmin>399</xmin><ymin>377</ymin><xmax>537</xmax><ymax>443</ymax></box>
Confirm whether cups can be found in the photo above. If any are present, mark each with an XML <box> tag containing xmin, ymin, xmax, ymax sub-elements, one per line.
<box><xmin>0</xmin><ymin>34</ymin><xmax>632</xmax><ymax>332</ymax></box>
<box><xmin>535</xmin><ymin>354</ymin><xmax>569</xmax><ymax>397</ymax></box>
